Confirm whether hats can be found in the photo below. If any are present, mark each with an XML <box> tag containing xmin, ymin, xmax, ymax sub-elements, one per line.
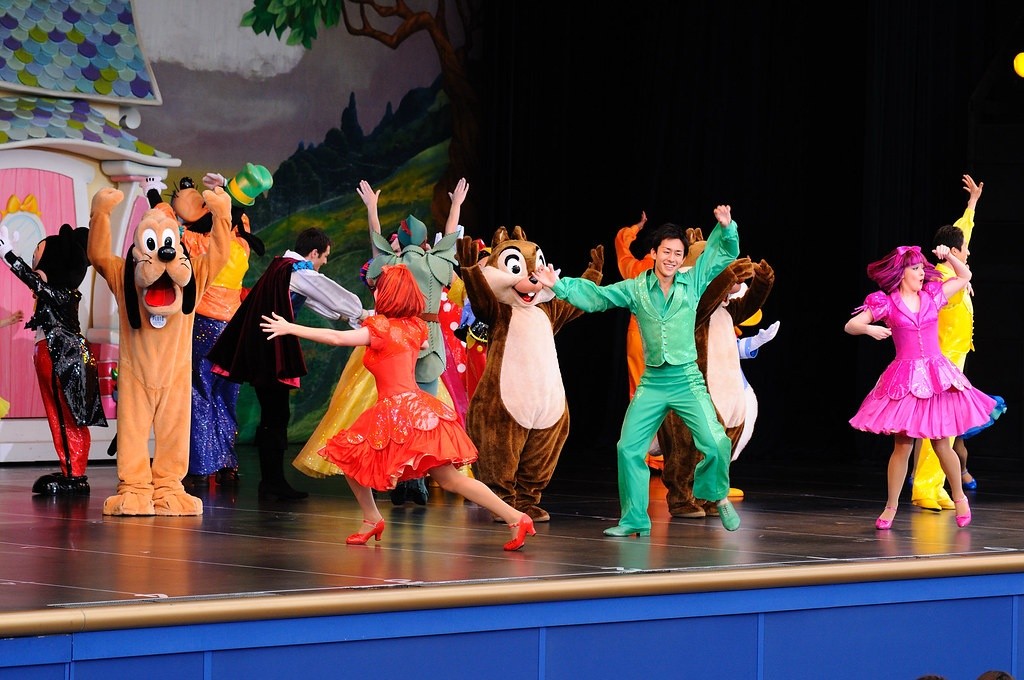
<box><xmin>397</xmin><ymin>215</ymin><xmax>428</xmax><ymax>248</ymax></box>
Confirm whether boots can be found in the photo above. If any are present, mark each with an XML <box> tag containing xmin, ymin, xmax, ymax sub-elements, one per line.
<box><xmin>259</xmin><ymin>425</ymin><xmax>309</xmax><ymax>499</ymax></box>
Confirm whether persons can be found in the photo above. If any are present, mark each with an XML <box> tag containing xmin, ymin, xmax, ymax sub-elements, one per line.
<box><xmin>0</xmin><ymin>311</ymin><xmax>24</xmax><ymax>421</ymax></box>
<box><xmin>845</xmin><ymin>173</ymin><xmax>1007</xmax><ymax>530</ymax></box>
<box><xmin>206</xmin><ymin>226</ymin><xmax>374</xmax><ymax>501</ymax></box>
<box><xmin>259</xmin><ymin>264</ymin><xmax>536</xmax><ymax>550</ymax></box>
<box><xmin>532</xmin><ymin>205</ymin><xmax>780</xmax><ymax>537</ymax></box>
<box><xmin>291</xmin><ymin>177</ymin><xmax>475</xmax><ymax>507</ymax></box>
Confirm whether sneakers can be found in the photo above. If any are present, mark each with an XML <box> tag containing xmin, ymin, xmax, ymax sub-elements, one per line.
<box><xmin>414</xmin><ymin>490</ymin><xmax>426</xmax><ymax>505</ymax></box>
<box><xmin>913</xmin><ymin>498</ymin><xmax>942</xmax><ymax>511</ymax></box>
<box><xmin>938</xmin><ymin>498</ymin><xmax>956</xmax><ymax>509</ymax></box>
<box><xmin>390</xmin><ymin>484</ymin><xmax>406</xmax><ymax>505</ymax></box>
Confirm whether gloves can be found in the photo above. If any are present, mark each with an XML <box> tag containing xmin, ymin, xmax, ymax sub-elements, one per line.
<box><xmin>749</xmin><ymin>321</ymin><xmax>780</xmax><ymax>351</ymax></box>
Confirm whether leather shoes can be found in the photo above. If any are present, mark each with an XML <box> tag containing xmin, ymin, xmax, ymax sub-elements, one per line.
<box><xmin>717</xmin><ymin>497</ymin><xmax>740</xmax><ymax>531</ymax></box>
<box><xmin>962</xmin><ymin>469</ymin><xmax>976</xmax><ymax>489</ymax></box>
<box><xmin>603</xmin><ymin>522</ymin><xmax>649</xmax><ymax>537</ymax></box>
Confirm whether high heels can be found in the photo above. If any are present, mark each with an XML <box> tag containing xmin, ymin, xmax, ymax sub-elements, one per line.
<box><xmin>876</xmin><ymin>506</ymin><xmax>897</xmax><ymax>529</ymax></box>
<box><xmin>503</xmin><ymin>514</ymin><xmax>536</xmax><ymax>550</ymax></box>
<box><xmin>346</xmin><ymin>518</ymin><xmax>386</xmax><ymax>544</ymax></box>
<box><xmin>954</xmin><ymin>496</ymin><xmax>971</xmax><ymax>527</ymax></box>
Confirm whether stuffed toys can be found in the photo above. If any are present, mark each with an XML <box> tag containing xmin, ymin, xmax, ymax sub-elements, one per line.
<box><xmin>87</xmin><ymin>163</ymin><xmax>273</xmax><ymax>516</ymax></box>
<box><xmin>0</xmin><ymin>223</ymin><xmax>108</xmax><ymax>498</ymax></box>
<box><xmin>656</xmin><ymin>227</ymin><xmax>775</xmax><ymax>517</ymax></box>
<box><xmin>454</xmin><ymin>224</ymin><xmax>604</xmax><ymax>522</ymax></box>
